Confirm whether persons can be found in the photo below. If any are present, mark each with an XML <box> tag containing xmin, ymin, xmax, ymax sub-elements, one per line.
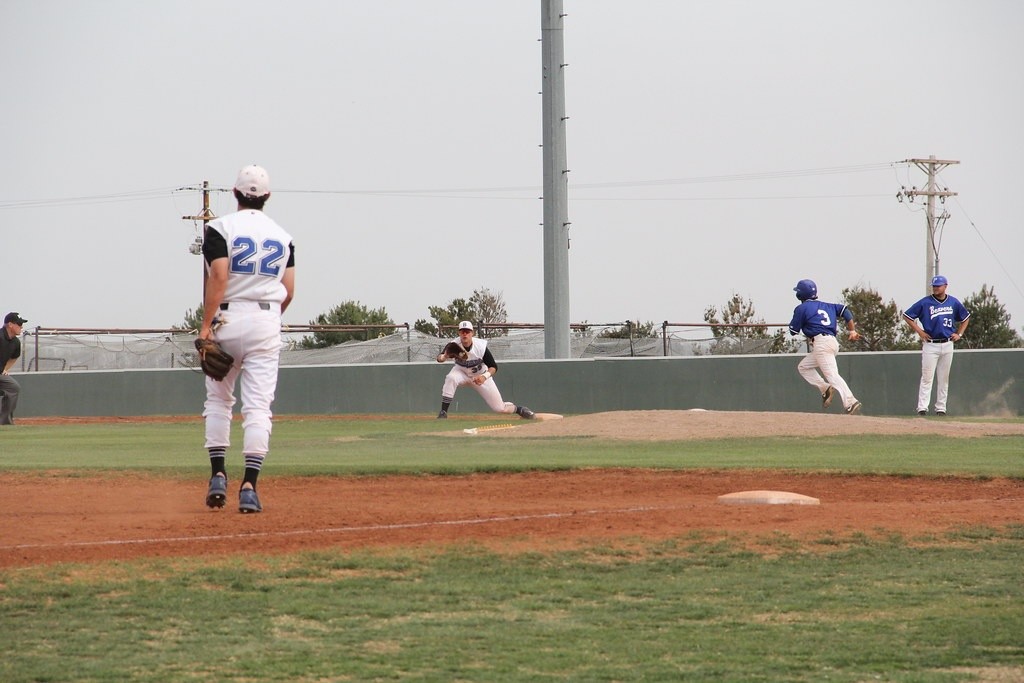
<box><xmin>437</xmin><ymin>320</ymin><xmax>534</xmax><ymax>420</ymax></box>
<box><xmin>902</xmin><ymin>276</ymin><xmax>971</xmax><ymax>416</ymax></box>
<box><xmin>789</xmin><ymin>280</ymin><xmax>861</xmax><ymax>414</ymax></box>
<box><xmin>197</xmin><ymin>166</ymin><xmax>294</xmax><ymax>513</ymax></box>
<box><xmin>0</xmin><ymin>312</ymin><xmax>28</xmax><ymax>425</ymax></box>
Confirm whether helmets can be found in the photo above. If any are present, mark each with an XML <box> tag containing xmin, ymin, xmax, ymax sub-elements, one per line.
<box><xmin>794</xmin><ymin>279</ymin><xmax>817</xmax><ymax>300</ymax></box>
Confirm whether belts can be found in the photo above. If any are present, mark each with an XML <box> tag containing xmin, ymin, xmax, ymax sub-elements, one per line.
<box><xmin>927</xmin><ymin>338</ymin><xmax>952</xmax><ymax>344</ymax></box>
<box><xmin>220</xmin><ymin>302</ymin><xmax>271</xmax><ymax>310</ymax></box>
<box><xmin>811</xmin><ymin>333</ymin><xmax>833</xmax><ymax>342</ymax></box>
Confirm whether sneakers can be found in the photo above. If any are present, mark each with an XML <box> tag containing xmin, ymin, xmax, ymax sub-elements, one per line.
<box><xmin>520</xmin><ymin>406</ymin><xmax>533</xmax><ymax>420</ymax></box>
<box><xmin>238</xmin><ymin>488</ymin><xmax>263</xmax><ymax>512</ymax></box>
<box><xmin>437</xmin><ymin>409</ymin><xmax>447</xmax><ymax>419</ymax></box>
<box><xmin>206</xmin><ymin>475</ymin><xmax>226</xmax><ymax>509</ymax></box>
<box><xmin>844</xmin><ymin>400</ymin><xmax>861</xmax><ymax>415</ymax></box>
<box><xmin>821</xmin><ymin>386</ymin><xmax>834</xmax><ymax>408</ymax></box>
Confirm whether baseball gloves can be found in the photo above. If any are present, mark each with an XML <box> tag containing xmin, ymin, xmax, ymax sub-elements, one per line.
<box><xmin>443</xmin><ymin>342</ymin><xmax>463</xmax><ymax>358</ymax></box>
<box><xmin>195</xmin><ymin>338</ymin><xmax>235</xmax><ymax>381</ymax></box>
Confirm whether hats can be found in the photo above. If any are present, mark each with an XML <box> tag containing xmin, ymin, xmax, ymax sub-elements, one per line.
<box><xmin>929</xmin><ymin>276</ymin><xmax>947</xmax><ymax>286</ymax></box>
<box><xmin>234</xmin><ymin>164</ymin><xmax>270</xmax><ymax>197</ymax></box>
<box><xmin>4</xmin><ymin>312</ymin><xmax>28</xmax><ymax>324</ymax></box>
<box><xmin>459</xmin><ymin>320</ymin><xmax>473</xmax><ymax>331</ymax></box>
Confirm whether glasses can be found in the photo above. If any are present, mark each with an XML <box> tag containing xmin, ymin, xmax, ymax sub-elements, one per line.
<box><xmin>13</xmin><ymin>322</ymin><xmax>22</xmax><ymax>326</ymax></box>
<box><xmin>459</xmin><ymin>330</ymin><xmax>471</xmax><ymax>335</ymax></box>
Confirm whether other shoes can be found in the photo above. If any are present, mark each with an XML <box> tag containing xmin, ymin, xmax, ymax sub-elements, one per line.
<box><xmin>919</xmin><ymin>411</ymin><xmax>926</xmax><ymax>416</ymax></box>
<box><xmin>937</xmin><ymin>412</ymin><xmax>945</xmax><ymax>416</ymax></box>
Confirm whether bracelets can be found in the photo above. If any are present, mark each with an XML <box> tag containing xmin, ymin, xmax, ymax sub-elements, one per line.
<box><xmin>958</xmin><ymin>333</ymin><xmax>962</xmax><ymax>337</ymax></box>
